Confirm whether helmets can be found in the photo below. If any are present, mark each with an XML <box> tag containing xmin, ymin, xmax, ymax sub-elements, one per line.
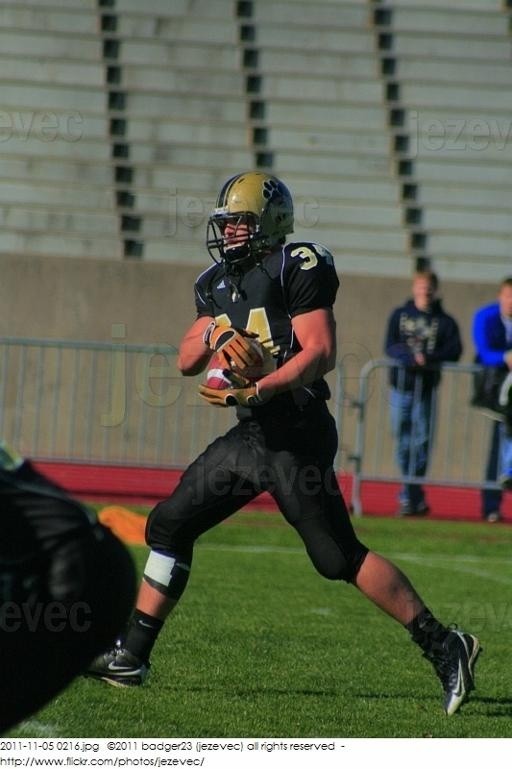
<box><xmin>211</xmin><ymin>172</ymin><xmax>295</xmax><ymax>264</ymax></box>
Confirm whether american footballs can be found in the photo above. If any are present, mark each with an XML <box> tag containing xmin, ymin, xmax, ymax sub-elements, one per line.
<box><xmin>203</xmin><ymin>335</ymin><xmax>265</xmax><ymax>391</ymax></box>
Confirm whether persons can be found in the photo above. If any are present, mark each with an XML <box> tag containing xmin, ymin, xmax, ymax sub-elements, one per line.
<box><xmin>81</xmin><ymin>167</ymin><xmax>484</xmax><ymax>723</ymax></box>
<box><xmin>380</xmin><ymin>265</ymin><xmax>464</xmax><ymax>521</ymax></box>
<box><xmin>469</xmin><ymin>277</ymin><xmax>512</xmax><ymax>522</ymax></box>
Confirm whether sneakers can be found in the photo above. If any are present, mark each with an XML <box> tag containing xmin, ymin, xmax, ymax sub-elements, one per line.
<box><xmin>78</xmin><ymin>647</ymin><xmax>150</xmax><ymax>690</ymax></box>
<box><xmin>435</xmin><ymin>627</ymin><xmax>482</xmax><ymax>718</ymax></box>
<box><xmin>482</xmin><ymin>510</ymin><xmax>502</xmax><ymax>523</ymax></box>
<box><xmin>399</xmin><ymin>501</ymin><xmax>429</xmax><ymax>517</ymax></box>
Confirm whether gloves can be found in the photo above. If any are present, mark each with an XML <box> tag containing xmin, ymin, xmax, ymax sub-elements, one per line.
<box><xmin>197</xmin><ymin>369</ymin><xmax>265</xmax><ymax>408</ymax></box>
<box><xmin>203</xmin><ymin>321</ymin><xmax>264</xmax><ymax>372</ymax></box>
